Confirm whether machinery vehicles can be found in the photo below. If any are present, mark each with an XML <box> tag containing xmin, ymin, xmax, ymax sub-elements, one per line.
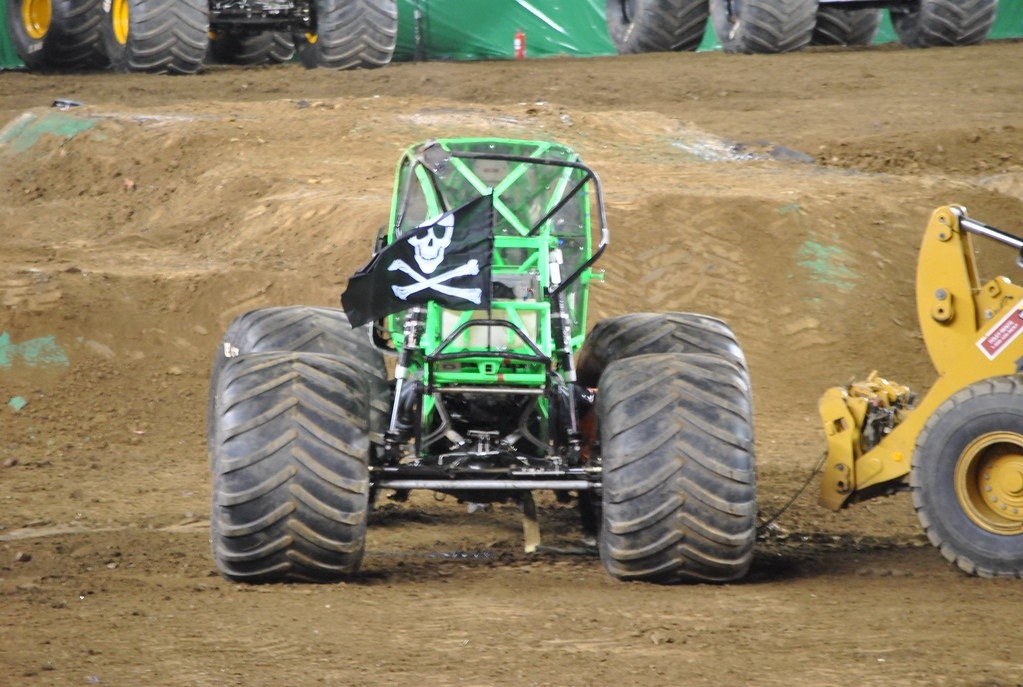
<box><xmin>818</xmin><ymin>205</ymin><xmax>1023</xmax><ymax>586</ymax></box>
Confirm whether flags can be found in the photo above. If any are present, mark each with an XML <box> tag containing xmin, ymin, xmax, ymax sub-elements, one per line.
<box><xmin>342</xmin><ymin>194</ymin><xmax>493</xmax><ymax>328</ymax></box>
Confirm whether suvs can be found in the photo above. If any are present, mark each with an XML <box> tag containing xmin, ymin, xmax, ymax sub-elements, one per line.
<box><xmin>603</xmin><ymin>1</ymin><xmax>1001</xmax><ymax>56</ymax></box>
<box><xmin>5</xmin><ymin>0</ymin><xmax>401</xmax><ymax>75</ymax></box>
<box><xmin>206</xmin><ymin>139</ymin><xmax>758</xmax><ymax>587</ymax></box>
